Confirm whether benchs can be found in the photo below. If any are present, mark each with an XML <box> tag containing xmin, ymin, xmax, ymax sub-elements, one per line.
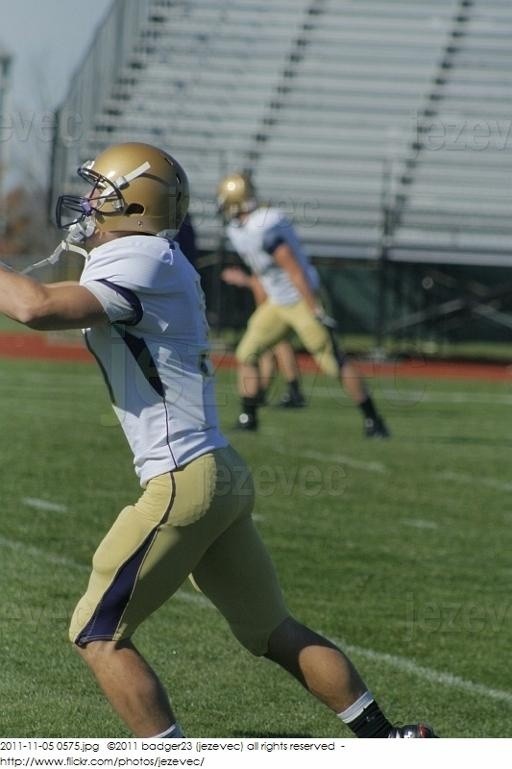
<box><xmin>46</xmin><ymin>1</ymin><xmax>510</xmax><ymax>265</ymax></box>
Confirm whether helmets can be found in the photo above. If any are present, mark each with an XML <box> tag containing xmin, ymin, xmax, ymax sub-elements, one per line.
<box><xmin>55</xmin><ymin>142</ymin><xmax>190</xmax><ymax>241</ymax></box>
<box><xmin>215</xmin><ymin>173</ymin><xmax>259</xmax><ymax>225</ymax></box>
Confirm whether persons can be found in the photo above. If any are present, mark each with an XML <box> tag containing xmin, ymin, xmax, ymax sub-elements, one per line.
<box><xmin>216</xmin><ymin>171</ymin><xmax>390</xmax><ymax>438</ymax></box>
<box><xmin>220</xmin><ymin>263</ymin><xmax>306</xmax><ymax>410</ymax></box>
<box><xmin>0</xmin><ymin>140</ymin><xmax>439</xmax><ymax>738</ymax></box>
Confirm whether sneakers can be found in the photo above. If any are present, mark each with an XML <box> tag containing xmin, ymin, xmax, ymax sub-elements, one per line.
<box><xmin>279</xmin><ymin>396</ymin><xmax>306</xmax><ymax>408</ymax></box>
<box><xmin>234</xmin><ymin>416</ymin><xmax>256</xmax><ymax>430</ymax></box>
<box><xmin>361</xmin><ymin>415</ymin><xmax>388</xmax><ymax>439</ymax></box>
